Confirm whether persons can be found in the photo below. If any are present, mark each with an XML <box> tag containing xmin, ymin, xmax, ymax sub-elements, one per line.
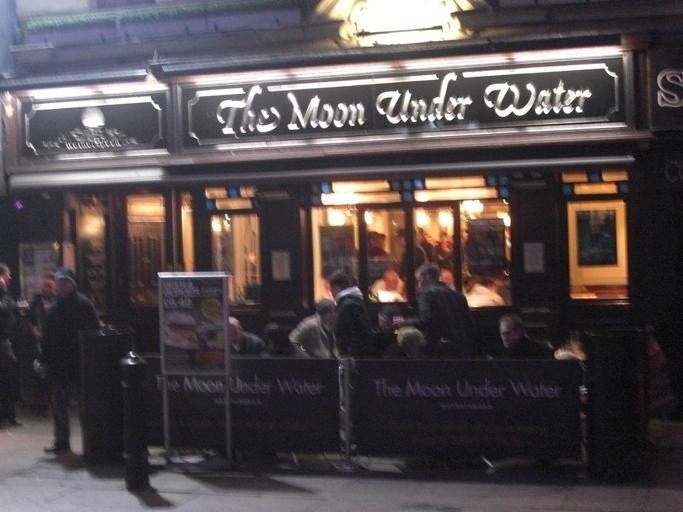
<box><xmin>35</xmin><ymin>267</ymin><xmax>99</xmax><ymax>454</ymax></box>
<box><xmin>228</xmin><ymin>225</ymin><xmax>614</xmax><ymax>468</ymax></box>
<box><xmin>0</xmin><ymin>260</ymin><xmax>30</xmax><ymax>431</ymax></box>
<box><xmin>579</xmin><ymin>212</ymin><xmax>614</xmax><ymax>263</ymax></box>
<box><xmin>23</xmin><ymin>271</ymin><xmax>56</xmax><ymax>421</ymax></box>
